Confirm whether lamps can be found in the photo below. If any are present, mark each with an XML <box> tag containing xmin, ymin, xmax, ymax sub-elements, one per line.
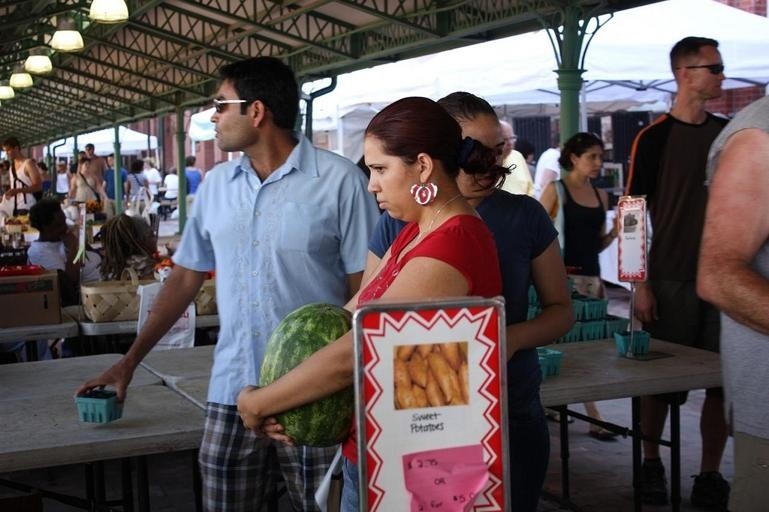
<box><xmin>0</xmin><ymin>86</ymin><xmax>14</xmax><ymax>99</ymax></box>
<box><xmin>89</xmin><ymin>0</ymin><xmax>128</xmax><ymax>21</ymax></box>
<box><xmin>49</xmin><ymin>31</ymin><xmax>84</xmax><ymax>51</ymax></box>
<box><xmin>9</xmin><ymin>73</ymin><xmax>33</xmax><ymax>87</ymax></box>
<box><xmin>25</xmin><ymin>55</ymin><xmax>52</xmax><ymax>73</ymax></box>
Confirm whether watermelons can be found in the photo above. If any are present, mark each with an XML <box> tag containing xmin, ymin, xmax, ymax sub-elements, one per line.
<box><xmin>258</xmin><ymin>302</ymin><xmax>353</xmax><ymax>448</ymax></box>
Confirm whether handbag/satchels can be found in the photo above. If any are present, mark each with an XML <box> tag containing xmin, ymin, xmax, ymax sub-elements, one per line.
<box><xmin>552</xmin><ymin>180</ymin><xmax>567</xmax><ymax>255</ymax></box>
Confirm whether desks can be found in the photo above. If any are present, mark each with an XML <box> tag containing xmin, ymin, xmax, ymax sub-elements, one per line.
<box><xmin>173</xmin><ymin>378</ymin><xmax>215</xmax><ymax>512</ymax></box>
<box><xmin>0</xmin><ymin>385</ymin><xmax>205</xmax><ymax>512</ymax></box>
<box><xmin>63</xmin><ymin>306</ymin><xmax>219</xmax><ymax>353</ymax></box>
<box><xmin>0</xmin><ymin>311</ymin><xmax>77</xmax><ymax>361</ymax></box>
<box><xmin>140</xmin><ymin>345</ymin><xmax>215</xmax><ymax>381</ymax></box>
<box><xmin>0</xmin><ymin>354</ymin><xmax>162</xmax><ymax>512</ymax></box>
<box><xmin>540</xmin><ymin>338</ymin><xmax>722</xmax><ymax>512</ymax></box>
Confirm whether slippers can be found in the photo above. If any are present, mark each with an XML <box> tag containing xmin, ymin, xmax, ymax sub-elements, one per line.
<box><xmin>586</xmin><ymin>421</ymin><xmax>620</xmax><ymax>441</ymax></box>
<box><xmin>544</xmin><ymin>409</ymin><xmax>574</xmax><ymax>425</ymax></box>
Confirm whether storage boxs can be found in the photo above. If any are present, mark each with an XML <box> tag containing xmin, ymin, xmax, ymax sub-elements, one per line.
<box><xmin>0</xmin><ymin>269</ymin><xmax>61</xmax><ymax>328</ymax></box>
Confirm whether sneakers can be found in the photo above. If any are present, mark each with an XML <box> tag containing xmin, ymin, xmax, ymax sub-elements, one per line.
<box><xmin>691</xmin><ymin>470</ymin><xmax>728</xmax><ymax>512</ymax></box>
<box><xmin>637</xmin><ymin>457</ymin><xmax>669</xmax><ymax>504</ymax></box>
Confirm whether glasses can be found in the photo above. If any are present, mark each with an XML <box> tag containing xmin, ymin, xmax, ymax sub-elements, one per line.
<box><xmin>212</xmin><ymin>98</ymin><xmax>246</xmax><ymax>113</ymax></box>
<box><xmin>674</xmin><ymin>64</ymin><xmax>725</xmax><ymax>74</ymax></box>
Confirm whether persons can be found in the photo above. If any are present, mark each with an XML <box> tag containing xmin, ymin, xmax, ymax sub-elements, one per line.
<box><xmin>238</xmin><ymin>97</ymin><xmax>504</xmax><ymax>512</ymax></box>
<box><xmin>25</xmin><ymin>201</ymin><xmax>100</xmax><ymax>307</ymax></box>
<box><xmin>100</xmin><ymin>153</ymin><xmax>131</xmax><ymax>217</ymax></box>
<box><xmin>364</xmin><ymin>91</ymin><xmax>575</xmax><ymax>512</ymax></box>
<box><xmin>37</xmin><ymin>162</ymin><xmax>51</xmax><ymax>197</ymax></box>
<box><xmin>699</xmin><ymin>82</ymin><xmax>767</xmax><ymax>512</ymax></box>
<box><xmin>620</xmin><ymin>37</ymin><xmax>734</xmax><ymax>512</ymax></box>
<box><xmin>123</xmin><ymin>160</ymin><xmax>148</xmax><ymax>212</ymax></box>
<box><xmin>68</xmin><ymin>158</ymin><xmax>107</xmax><ymax>251</ymax></box>
<box><xmin>488</xmin><ymin>120</ymin><xmax>533</xmax><ymax>195</ymax></box>
<box><xmin>541</xmin><ymin>132</ymin><xmax>621</xmax><ymax>441</ymax></box>
<box><xmin>143</xmin><ymin>159</ymin><xmax>164</xmax><ymax>204</ymax></box>
<box><xmin>1</xmin><ymin>136</ymin><xmax>42</xmax><ymax>216</ymax></box>
<box><xmin>82</xmin><ymin>145</ymin><xmax>109</xmax><ymax>194</ymax></box>
<box><xmin>75</xmin><ymin>56</ymin><xmax>384</xmax><ymax>511</ymax></box>
<box><xmin>53</xmin><ymin>159</ymin><xmax>71</xmax><ymax>197</ymax></box>
<box><xmin>184</xmin><ymin>156</ymin><xmax>202</xmax><ymax>212</ymax></box>
<box><xmin>164</xmin><ymin>167</ymin><xmax>182</xmax><ymax>205</ymax></box>
<box><xmin>101</xmin><ymin>212</ymin><xmax>169</xmax><ymax>281</ymax></box>
<box><xmin>532</xmin><ymin>141</ymin><xmax>563</xmax><ymax>207</ymax></box>
